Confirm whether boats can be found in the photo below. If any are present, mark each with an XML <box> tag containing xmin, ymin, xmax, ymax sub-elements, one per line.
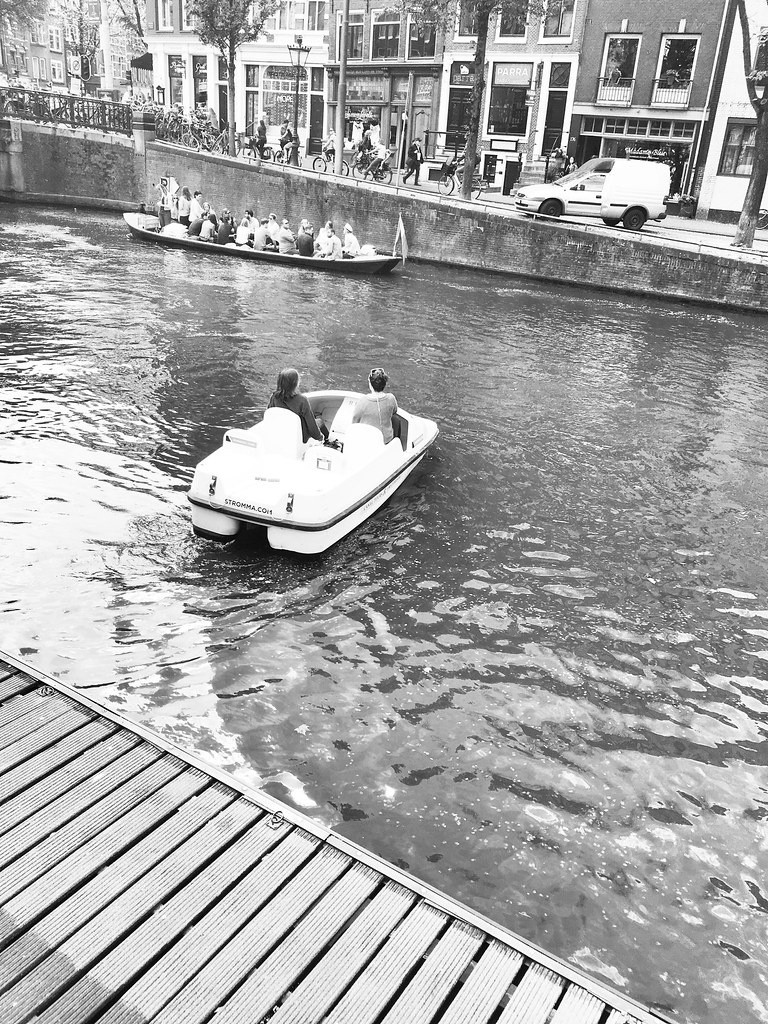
<box><xmin>183</xmin><ymin>390</ymin><xmax>440</xmax><ymax>559</ymax></box>
<box><xmin>121</xmin><ymin>211</ymin><xmax>404</xmax><ymax>275</ymax></box>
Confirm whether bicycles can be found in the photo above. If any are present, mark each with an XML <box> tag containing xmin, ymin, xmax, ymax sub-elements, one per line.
<box><xmin>437</xmin><ymin>160</ymin><xmax>482</xmax><ymax>200</ymax></box>
<box><xmin>155</xmin><ymin>113</ymin><xmax>302</xmax><ymax>168</ymax></box>
<box><xmin>313</xmin><ymin>147</ymin><xmax>393</xmax><ymax>184</ymax></box>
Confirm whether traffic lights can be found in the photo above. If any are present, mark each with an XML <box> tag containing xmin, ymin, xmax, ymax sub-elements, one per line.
<box><xmin>80</xmin><ymin>55</ymin><xmax>91</xmax><ymax>82</ymax></box>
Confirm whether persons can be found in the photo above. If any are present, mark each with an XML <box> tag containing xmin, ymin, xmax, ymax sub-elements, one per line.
<box><xmin>154</xmin><ymin>174</ymin><xmax>360</xmax><ymax>261</ymax></box>
<box><xmin>124</xmin><ymin>86</ymin><xmax>578</xmax><ymax>191</ymax></box>
<box><xmin>264</xmin><ymin>367</ymin><xmax>339</xmax><ymax>449</ymax></box>
<box><xmin>351</xmin><ymin>367</ymin><xmax>401</xmax><ymax>444</ymax></box>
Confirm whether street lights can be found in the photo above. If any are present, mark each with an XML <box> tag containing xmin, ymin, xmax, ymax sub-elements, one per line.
<box><xmin>286</xmin><ymin>37</ymin><xmax>312</xmax><ymax>166</ymax></box>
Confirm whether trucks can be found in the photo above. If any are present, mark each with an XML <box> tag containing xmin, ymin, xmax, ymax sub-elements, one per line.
<box><xmin>515</xmin><ymin>157</ymin><xmax>673</xmax><ymax>231</ymax></box>
